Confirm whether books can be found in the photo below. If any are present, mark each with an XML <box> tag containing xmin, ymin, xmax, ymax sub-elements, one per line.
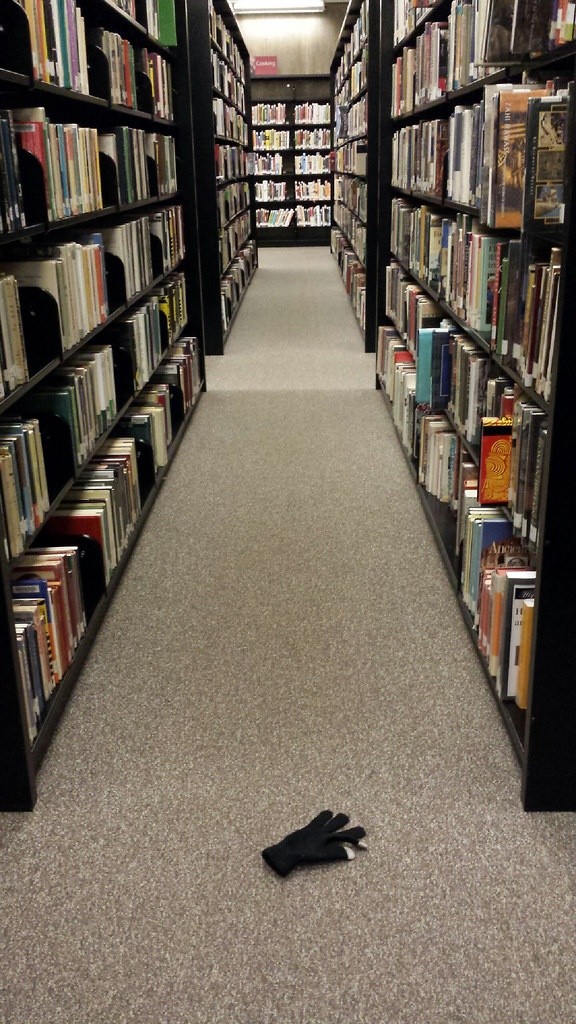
<box><xmin>390</xmin><ymin>0</ymin><xmax>575</xmax><ymax>120</ymax></box>
<box><xmin>389</xmin><ymin>196</ymin><xmax>563</xmax><ymax>407</ymax></box>
<box><xmin>2</xmin><ymin>205</ymin><xmax>187</xmax><ymax>401</ymax></box>
<box><xmin>3</xmin><ymin>338</ymin><xmax>208</xmax><ymax>749</ymax></box>
<box><xmin>1</xmin><ymin>104</ymin><xmax>179</xmax><ymax>229</ymax></box>
<box><xmin>206</xmin><ymin>2</ymin><xmax>367</xmax><ymax>336</ymax></box>
<box><xmin>385</xmin><ymin>257</ymin><xmax>550</xmax><ymax>554</ymax></box>
<box><xmin>2</xmin><ymin>271</ymin><xmax>192</xmax><ymax>561</ymax></box>
<box><xmin>1</xmin><ymin>0</ymin><xmax>177</xmax><ymax>119</ymax></box>
<box><xmin>391</xmin><ymin>76</ymin><xmax>572</xmax><ymax>233</ymax></box>
<box><xmin>376</xmin><ymin>324</ymin><xmax>550</xmax><ymax>712</ymax></box>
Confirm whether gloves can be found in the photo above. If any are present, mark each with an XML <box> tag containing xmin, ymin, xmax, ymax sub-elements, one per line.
<box><xmin>261</xmin><ymin>810</ymin><xmax>368</xmax><ymax>877</ymax></box>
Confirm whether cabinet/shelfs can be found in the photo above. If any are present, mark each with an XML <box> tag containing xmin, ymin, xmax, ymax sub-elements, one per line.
<box><xmin>187</xmin><ymin>0</ymin><xmax>259</xmax><ymax>356</ymax></box>
<box><xmin>0</xmin><ymin>0</ymin><xmax>207</xmax><ymax>811</ymax></box>
<box><xmin>330</xmin><ymin>0</ymin><xmax>375</xmax><ymax>354</ymax></box>
<box><xmin>250</xmin><ymin>73</ymin><xmax>330</xmax><ymax>247</ymax></box>
<box><xmin>375</xmin><ymin>0</ymin><xmax>576</xmax><ymax>811</ymax></box>
